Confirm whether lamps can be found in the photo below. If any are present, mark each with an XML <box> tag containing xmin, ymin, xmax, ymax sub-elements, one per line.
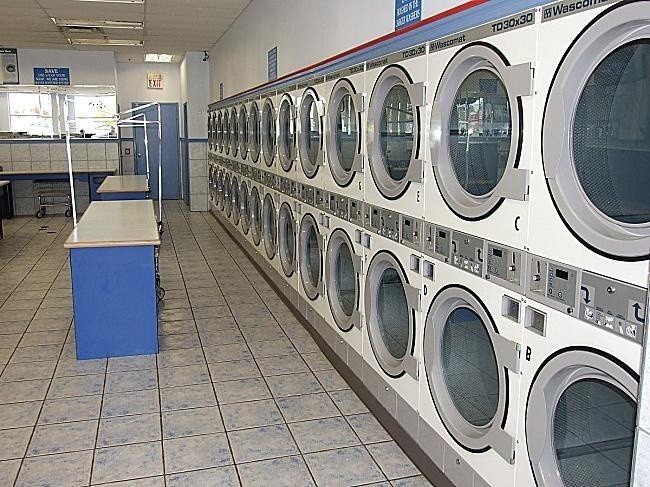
<box><xmin>50</xmin><ymin>0</ymin><xmax>146</xmax><ymax>47</ymax></box>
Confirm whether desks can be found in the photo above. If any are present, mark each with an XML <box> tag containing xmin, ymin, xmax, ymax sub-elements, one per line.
<box><xmin>1</xmin><ymin>168</ymin><xmax>118</xmax><ymax>202</ymax></box>
<box><xmin>63</xmin><ymin>198</ymin><xmax>163</xmax><ymax>362</ymax></box>
<box><xmin>96</xmin><ymin>174</ymin><xmax>148</xmax><ymax>200</ymax></box>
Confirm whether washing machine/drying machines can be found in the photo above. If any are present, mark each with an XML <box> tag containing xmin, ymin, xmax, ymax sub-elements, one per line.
<box><xmin>206</xmin><ymin>1</ymin><xmax>649</xmax><ymax>487</ymax></box>
